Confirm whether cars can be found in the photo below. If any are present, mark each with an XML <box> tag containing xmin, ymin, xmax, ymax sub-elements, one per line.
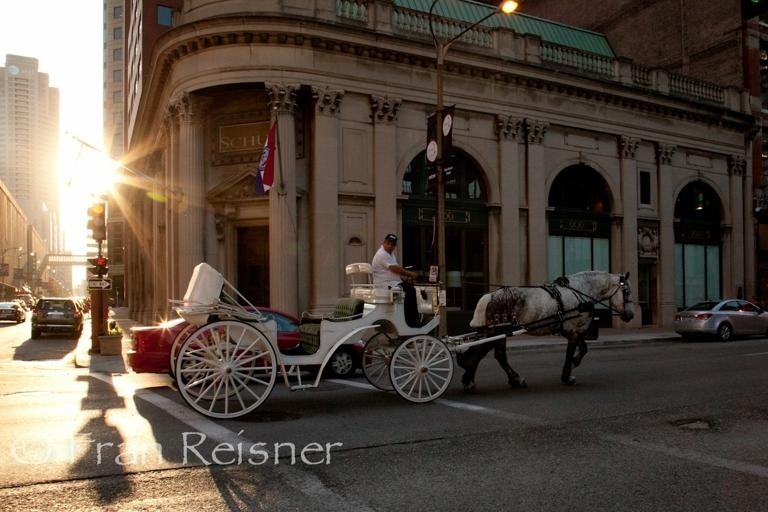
<box><xmin>0</xmin><ymin>294</ymin><xmax>92</xmax><ymax>340</ymax></box>
<box><xmin>671</xmin><ymin>298</ymin><xmax>768</xmax><ymax>342</ymax></box>
<box><xmin>127</xmin><ymin>306</ymin><xmax>373</xmax><ymax>385</ymax></box>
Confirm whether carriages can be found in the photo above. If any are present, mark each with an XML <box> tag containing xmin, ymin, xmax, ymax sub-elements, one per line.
<box><xmin>170</xmin><ymin>263</ymin><xmax>634</xmax><ymax>419</ymax></box>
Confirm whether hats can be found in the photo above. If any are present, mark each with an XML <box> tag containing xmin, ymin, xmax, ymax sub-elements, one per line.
<box><xmin>384</xmin><ymin>233</ymin><xmax>399</xmax><ymax>244</ymax></box>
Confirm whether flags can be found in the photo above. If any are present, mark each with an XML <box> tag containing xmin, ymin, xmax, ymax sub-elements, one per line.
<box><xmin>255</xmin><ymin>121</ymin><xmax>275</xmax><ymax>194</ymax></box>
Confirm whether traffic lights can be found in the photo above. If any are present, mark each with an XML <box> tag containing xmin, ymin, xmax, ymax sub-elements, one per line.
<box><xmin>87</xmin><ymin>201</ymin><xmax>105</xmax><ymax>240</ymax></box>
<box><xmin>88</xmin><ymin>256</ymin><xmax>109</xmax><ymax>275</ymax></box>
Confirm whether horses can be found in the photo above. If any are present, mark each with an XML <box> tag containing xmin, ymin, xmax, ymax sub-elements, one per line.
<box><xmin>460</xmin><ymin>270</ymin><xmax>635</xmax><ymax>396</ymax></box>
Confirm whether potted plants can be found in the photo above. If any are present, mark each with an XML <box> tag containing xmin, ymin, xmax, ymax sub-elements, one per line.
<box><xmin>98</xmin><ymin>320</ymin><xmax>124</xmax><ymax>357</ymax></box>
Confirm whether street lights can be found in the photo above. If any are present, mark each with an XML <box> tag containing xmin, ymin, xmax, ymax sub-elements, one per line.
<box><xmin>2</xmin><ymin>246</ymin><xmax>23</xmax><ymax>286</ymax></box>
<box><xmin>27</xmin><ymin>253</ymin><xmax>55</xmax><ymax>286</ymax></box>
<box><xmin>17</xmin><ymin>251</ymin><xmax>35</xmax><ymax>285</ymax></box>
<box><xmin>429</xmin><ymin>0</ymin><xmax>519</xmax><ymax>334</ymax></box>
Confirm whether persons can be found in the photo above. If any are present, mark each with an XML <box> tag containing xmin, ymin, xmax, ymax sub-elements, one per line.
<box><xmin>371</xmin><ymin>234</ymin><xmax>423</xmax><ymax>327</ymax></box>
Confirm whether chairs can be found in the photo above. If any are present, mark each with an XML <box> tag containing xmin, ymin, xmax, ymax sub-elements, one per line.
<box><xmin>298</xmin><ymin>297</ymin><xmax>364</xmax><ymax>352</ymax></box>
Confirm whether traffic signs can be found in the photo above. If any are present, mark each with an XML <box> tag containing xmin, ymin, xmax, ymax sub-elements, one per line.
<box><xmin>88</xmin><ymin>278</ymin><xmax>112</xmax><ymax>290</ymax></box>
